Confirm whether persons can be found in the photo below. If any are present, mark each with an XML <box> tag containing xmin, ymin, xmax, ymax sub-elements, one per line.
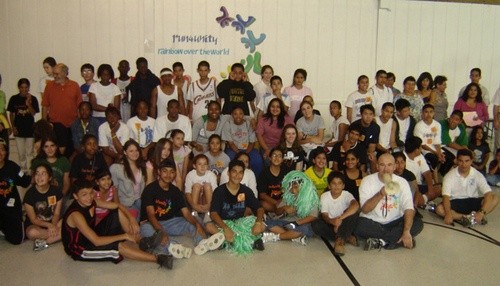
<box><xmin>248</xmin><ymin>64</ymin><xmax>424</xmax><ymax>257</ymax></box>
<box><xmin>384</xmin><ymin>67</ymin><xmax>500</xmax><ymax>228</ymax></box>
<box><xmin>0</xmin><ymin>55</ymin><xmax>173</xmax><ymax>270</ymax></box>
<box><xmin>124</xmin><ymin>57</ymin><xmax>266</xmax><ymax>260</ymax></box>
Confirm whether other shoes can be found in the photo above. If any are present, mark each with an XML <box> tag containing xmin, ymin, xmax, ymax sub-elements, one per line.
<box><xmin>167</xmin><ymin>241</ymin><xmax>192</xmax><ymax>259</ymax></box>
<box><xmin>333</xmin><ymin>236</ymin><xmax>345</xmax><ymax>256</ymax></box>
<box><xmin>460</xmin><ymin>210</ymin><xmax>488</xmax><ymax>228</ymax></box>
<box><xmin>192</xmin><ymin>210</ymin><xmax>202</xmax><ymax>224</ymax></box>
<box><xmin>261</xmin><ymin>232</ymin><xmax>281</xmax><ymax>244</ymax></box>
<box><xmin>139</xmin><ymin>229</ymin><xmax>162</xmax><ymax>254</ymax></box>
<box><xmin>421</xmin><ymin>200</ymin><xmax>436</xmax><ymax>212</ymax></box>
<box><xmin>396</xmin><ymin>237</ymin><xmax>417</xmax><ymax>249</ymax></box>
<box><xmin>346</xmin><ymin>233</ymin><xmax>360</xmax><ymax>247</ymax></box>
<box><xmin>364</xmin><ymin>237</ymin><xmax>383</xmax><ymax>253</ymax></box>
<box><xmin>193</xmin><ymin>232</ymin><xmax>225</xmax><ymax>256</ymax></box>
<box><xmin>203</xmin><ymin>211</ymin><xmax>212</xmax><ymax>224</ymax></box>
<box><xmin>33</xmin><ymin>239</ymin><xmax>49</xmax><ymax>251</ymax></box>
<box><xmin>290</xmin><ymin>234</ymin><xmax>309</xmax><ymax>246</ymax></box>
<box><xmin>157</xmin><ymin>254</ymin><xmax>173</xmax><ymax>270</ymax></box>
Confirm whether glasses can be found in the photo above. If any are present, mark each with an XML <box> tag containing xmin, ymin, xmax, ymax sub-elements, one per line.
<box><xmin>271</xmin><ymin>154</ymin><xmax>283</xmax><ymax>158</ymax></box>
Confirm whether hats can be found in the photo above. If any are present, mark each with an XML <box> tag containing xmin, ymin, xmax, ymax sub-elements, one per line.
<box><xmin>159</xmin><ymin>159</ymin><xmax>176</xmax><ymax>170</ymax></box>
<box><xmin>0</xmin><ymin>138</ymin><xmax>7</xmax><ymax>145</ymax></box>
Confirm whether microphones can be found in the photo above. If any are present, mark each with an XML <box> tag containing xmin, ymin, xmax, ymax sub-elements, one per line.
<box><xmin>383</xmin><ymin>172</ymin><xmax>393</xmax><ymax>196</ymax></box>
